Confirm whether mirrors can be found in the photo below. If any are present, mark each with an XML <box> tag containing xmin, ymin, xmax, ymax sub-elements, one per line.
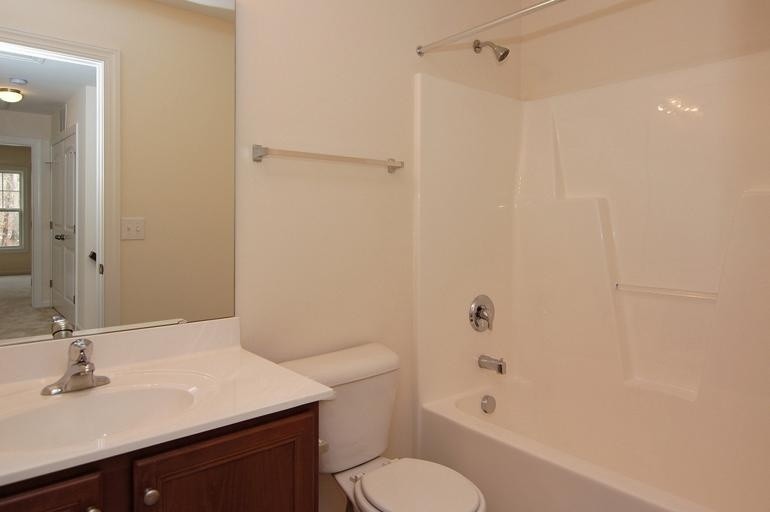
<box><xmin>0</xmin><ymin>0</ymin><xmax>237</xmax><ymax>347</ymax></box>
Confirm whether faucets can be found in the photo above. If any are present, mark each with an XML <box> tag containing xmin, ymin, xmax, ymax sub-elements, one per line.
<box><xmin>50</xmin><ymin>314</ymin><xmax>77</xmax><ymax>340</ymax></box>
<box><xmin>476</xmin><ymin>350</ymin><xmax>509</xmax><ymax>376</ymax></box>
<box><xmin>41</xmin><ymin>338</ymin><xmax>111</xmax><ymax>396</ymax></box>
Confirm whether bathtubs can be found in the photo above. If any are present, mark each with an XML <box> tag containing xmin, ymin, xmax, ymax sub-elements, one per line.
<box><xmin>419</xmin><ymin>385</ymin><xmax>770</xmax><ymax>512</ymax></box>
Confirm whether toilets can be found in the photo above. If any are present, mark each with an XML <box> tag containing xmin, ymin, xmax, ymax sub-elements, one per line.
<box><xmin>275</xmin><ymin>342</ymin><xmax>489</xmax><ymax>512</ymax></box>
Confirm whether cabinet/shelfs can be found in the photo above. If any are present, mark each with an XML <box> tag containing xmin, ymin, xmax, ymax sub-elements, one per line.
<box><xmin>0</xmin><ymin>402</ymin><xmax>319</xmax><ymax>512</ymax></box>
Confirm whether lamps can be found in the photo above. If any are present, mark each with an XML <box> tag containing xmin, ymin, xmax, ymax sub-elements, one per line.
<box><xmin>0</xmin><ymin>88</ymin><xmax>23</xmax><ymax>103</ymax></box>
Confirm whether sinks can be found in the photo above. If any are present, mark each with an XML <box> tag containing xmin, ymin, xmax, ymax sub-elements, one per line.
<box><xmin>0</xmin><ymin>369</ymin><xmax>221</xmax><ymax>474</ymax></box>
